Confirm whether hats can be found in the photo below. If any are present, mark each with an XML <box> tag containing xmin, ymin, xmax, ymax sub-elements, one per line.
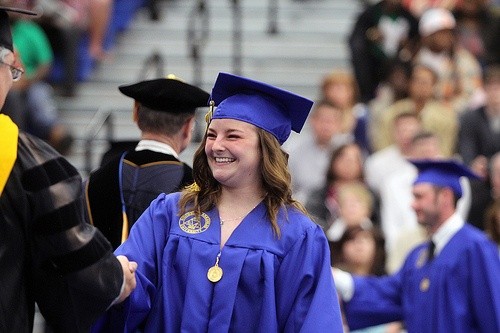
<box><xmin>184</xmin><ymin>72</ymin><xmax>314</xmax><ymax>194</ymax></box>
<box><xmin>408</xmin><ymin>158</ymin><xmax>483</xmax><ymax>198</ymax></box>
<box><xmin>0</xmin><ymin>7</ymin><xmax>38</xmax><ymax>54</ymax></box>
<box><xmin>419</xmin><ymin>7</ymin><xmax>457</xmax><ymax>38</ymax></box>
<box><xmin>118</xmin><ymin>78</ymin><xmax>212</xmax><ymax>117</ymax></box>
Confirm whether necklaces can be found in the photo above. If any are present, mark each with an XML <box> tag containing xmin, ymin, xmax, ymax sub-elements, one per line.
<box><xmin>220</xmin><ymin>217</ymin><xmax>242</xmax><ymax>225</ymax></box>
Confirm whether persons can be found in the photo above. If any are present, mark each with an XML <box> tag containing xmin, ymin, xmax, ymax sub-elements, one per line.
<box><xmin>287</xmin><ymin>0</ymin><xmax>500</xmax><ymax>276</ymax></box>
<box><xmin>0</xmin><ymin>6</ymin><xmax>137</xmax><ymax>333</ymax></box>
<box><xmin>91</xmin><ymin>72</ymin><xmax>344</xmax><ymax>333</ymax></box>
<box><xmin>82</xmin><ymin>78</ymin><xmax>210</xmax><ymax>250</ymax></box>
<box><xmin>0</xmin><ymin>0</ymin><xmax>143</xmax><ymax>153</ymax></box>
<box><xmin>331</xmin><ymin>159</ymin><xmax>500</xmax><ymax>332</ymax></box>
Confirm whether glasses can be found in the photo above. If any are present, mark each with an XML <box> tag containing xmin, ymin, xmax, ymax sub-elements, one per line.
<box><xmin>0</xmin><ymin>57</ymin><xmax>24</xmax><ymax>82</ymax></box>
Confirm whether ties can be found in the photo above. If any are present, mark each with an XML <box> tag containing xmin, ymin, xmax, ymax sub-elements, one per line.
<box><xmin>427</xmin><ymin>241</ymin><xmax>435</xmax><ymax>264</ymax></box>
